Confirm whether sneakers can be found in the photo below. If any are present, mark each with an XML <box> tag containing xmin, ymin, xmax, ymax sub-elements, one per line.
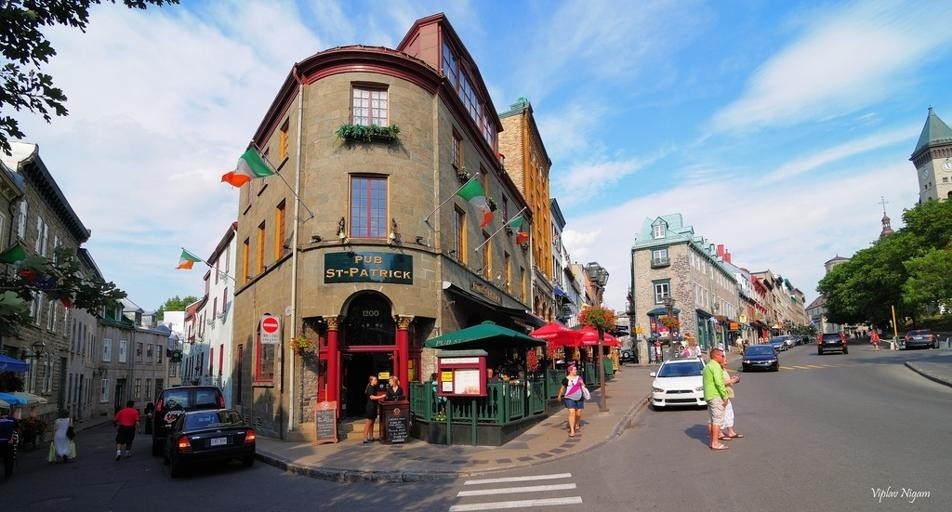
<box><xmin>569</xmin><ymin>432</ymin><xmax>575</xmax><ymax>436</ymax></box>
<box><xmin>115</xmin><ymin>452</ymin><xmax>122</xmax><ymax>461</ymax></box>
<box><xmin>58</xmin><ymin>455</ymin><xmax>69</xmax><ymax>463</ymax></box>
<box><xmin>124</xmin><ymin>452</ymin><xmax>134</xmax><ymax>457</ymax></box>
<box><xmin>363</xmin><ymin>438</ymin><xmax>380</xmax><ymax>443</ymax></box>
<box><xmin>575</xmin><ymin>424</ymin><xmax>580</xmax><ymax>430</ymax></box>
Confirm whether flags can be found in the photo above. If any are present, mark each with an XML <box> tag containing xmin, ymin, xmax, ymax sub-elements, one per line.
<box><xmin>221</xmin><ymin>146</ymin><xmax>274</xmax><ymax>189</ymax></box>
<box><xmin>175</xmin><ymin>250</ymin><xmax>201</xmax><ymax>269</ymax></box>
<box><xmin>457</xmin><ymin>178</ymin><xmax>494</xmax><ymax>228</ymax></box>
<box><xmin>509</xmin><ymin>214</ymin><xmax>530</xmax><ymax>245</ymax></box>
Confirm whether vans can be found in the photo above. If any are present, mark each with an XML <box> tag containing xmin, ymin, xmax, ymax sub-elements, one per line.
<box><xmin>145</xmin><ymin>385</ymin><xmax>227</xmax><ymax>456</ymax></box>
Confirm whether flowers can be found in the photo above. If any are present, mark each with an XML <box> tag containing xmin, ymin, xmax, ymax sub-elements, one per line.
<box><xmin>285</xmin><ymin>336</ymin><xmax>318</xmax><ymax>356</ymax></box>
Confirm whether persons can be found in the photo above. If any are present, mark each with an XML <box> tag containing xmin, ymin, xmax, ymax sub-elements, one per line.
<box><xmin>384</xmin><ymin>376</ymin><xmax>403</xmax><ymax>400</ymax></box>
<box><xmin>557</xmin><ymin>363</ymin><xmax>585</xmax><ymax>437</ymax></box>
<box><xmin>718</xmin><ymin>355</ymin><xmax>744</xmax><ymax>441</ymax></box>
<box><xmin>52</xmin><ymin>410</ymin><xmax>75</xmax><ymax>462</ymax></box>
<box><xmin>430</xmin><ymin>358</ymin><xmax>530</xmax><ymax>410</ymax></box>
<box><xmin>113</xmin><ymin>401</ymin><xmax>141</xmax><ymax>460</ymax></box>
<box><xmin>701</xmin><ymin>348</ymin><xmax>729</xmax><ymax>449</ymax></box>
<box><xmin>853</xmin><ymin>326</ymin><xmax>879</xmax><ymax>349</ymax></box>
<box><xmin>735</xmin><ymin>335</ymin><xmax>792</xmax><ymax>355</ymax></box>
<box><xmin>363</xmin><ymin>375</ymin><xmax>387</xmax><ymax>443</ymax></box>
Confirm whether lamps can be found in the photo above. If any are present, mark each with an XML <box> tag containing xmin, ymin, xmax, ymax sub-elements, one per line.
<box><xmin>337</xmin><ymin>213</ymin><xmax>348</xmax><ymax>242</ymax></box>
<box><xmin>481</xmin><ymin>261</ymin><xmax>492</xmax><ymax>277</ymax></box>
<box><xmin>500</xmin><ymin>276</ymin><xmax>513</xmax><ymax>288</ymax></box>
<box><xmin>490</xmin><ymin>270</ymin><xmax>501</xmax><ymax>287</ymax></box>
<box><xmin>387</xmin><ymin>217</ymin><xmax>402</xmax><ymax>244</ymax></box>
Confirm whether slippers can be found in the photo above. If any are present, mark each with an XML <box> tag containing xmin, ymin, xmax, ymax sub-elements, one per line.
<box><xmin>710</xmin><ymin>442</ymin><xmax>729</xmax><ymax>449</ymax></box>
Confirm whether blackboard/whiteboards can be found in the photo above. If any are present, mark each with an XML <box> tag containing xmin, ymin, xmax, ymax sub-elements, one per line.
<box><xmin>387</xmin><ymin>416</ymin><xmax>407</xmax><ymax>443</ymax></box>
<box><xmin>314</xmin><ymin>401</ymin><xmax>337</xmax><ymax>441</ymax></box>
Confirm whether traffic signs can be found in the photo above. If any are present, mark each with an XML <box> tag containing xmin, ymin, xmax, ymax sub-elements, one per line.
<box><xmin>616</xmin><ymin>325</ymin><xmax>628</xmax><ymax>330</ymax></box>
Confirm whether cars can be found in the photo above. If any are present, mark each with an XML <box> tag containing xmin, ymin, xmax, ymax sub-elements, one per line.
<box><xmin>648</xmin><ymin>358</ymin><xmax>708</xmax><ymax>409</ymax></box>
<box><xmin>769</xmin><ymin>333</ymin><xmax>817</xmax><ymax>352</ymax></box>
<box><xmin>903</xmin><ymin>328</ymin><xmax>941</xmax><ymax>350</ymax></box>
<box><xmin>739</xmin><ymin>343</ymin><xmax>781</xmax><ymax>372</ymax></box>
<box><xmin>164</xmin><ymin>407</ymin><xmax>257</xmax><ymax>478</ymax></box>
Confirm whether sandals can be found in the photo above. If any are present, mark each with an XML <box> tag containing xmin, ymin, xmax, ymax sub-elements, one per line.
<box><xmin>728</xmin><ymin>433</ymin><xmax>744</xmax><ymax>438</ymax></box>
<box><xmin>718</xmin><ymin>433</ymin><xmax>731</xmax><ymax>440</ymax></box>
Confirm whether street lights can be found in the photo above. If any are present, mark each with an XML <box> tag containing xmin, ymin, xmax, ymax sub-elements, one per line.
<box><xmin>664</xmin><ymin>295</ymin><xmax>677</xmax><ymax>359</ymax></box>
<box><xmin>585</xmin><ymin>261</ymin><xmax>609</xmax><ymax>412</ymax></box>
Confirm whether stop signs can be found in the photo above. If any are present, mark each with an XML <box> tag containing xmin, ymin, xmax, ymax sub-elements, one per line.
<box><xmin>261</xmin><ymin>316</ymin><xmax>278</xmax><ymax>334</ymax></box>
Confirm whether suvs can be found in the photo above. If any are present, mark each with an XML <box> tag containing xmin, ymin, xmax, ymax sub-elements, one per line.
<box><xmin>816</xmin><ymin>330</ymin><xmax>849</xmax><ymax>357</ymax></box>
<box><xmin>619</xmin><ymin>347</ymin><xmax>635</xmax><ymax>364</ymax></box>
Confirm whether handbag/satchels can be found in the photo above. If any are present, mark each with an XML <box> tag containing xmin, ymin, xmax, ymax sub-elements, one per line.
<box><xmin>581</xmin><ymin>385</ymin><xmax>591</xmax><ymax>401</ymax></box>
<box><xmin>67</xmin><ymin>427</ymin><xmax>75</xmax><ymax>440</ymax></box>
<box><xmin>48</xmin><ymin>441</ymin><xmax>57</xmax><ymax>462</ymax></box>
<box><xmin>70</xmin><ymin>442</ymin><xmax>77</xmax><ymax>459</ymax></box>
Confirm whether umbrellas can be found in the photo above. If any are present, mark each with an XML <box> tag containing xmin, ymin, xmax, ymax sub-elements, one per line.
<box><xmin>529</xmin><ymin>319</ymin><xmax>584</xmax><ymax>369</ymax></box>
<box><xmin>421</xmin><ymin>318</ymin><xmax>546</xmax><ymax>353</ymax></box>
<box><xmin>573</xmin><ymin>325</ymin><xmax>622</xmax><ymax>347</ymax></box>
